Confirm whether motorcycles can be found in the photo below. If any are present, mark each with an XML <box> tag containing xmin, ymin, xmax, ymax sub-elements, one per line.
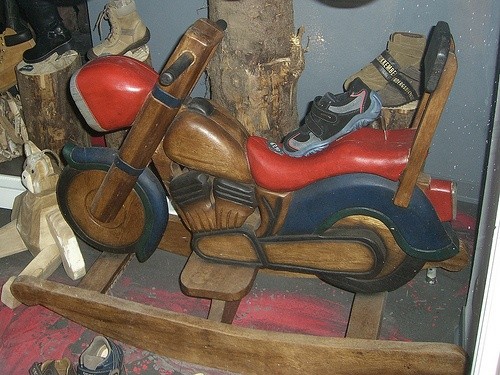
<box><xmin>54</xmin><ymin>18</ymin><xmax>461</xmax><ymax>296</ymax></box>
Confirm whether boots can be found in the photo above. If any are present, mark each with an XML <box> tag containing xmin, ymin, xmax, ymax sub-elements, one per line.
<box><xmin>344</xmin><ymin>34</ymin><xmax>426</xmax><ymax>95</ymax></box>
<box><xmin>87</xmin><ymin>0</ymin><xmax>151</xmax><ymax>63</ymax></box>
<box><xmin>21</xmin><ymin>1</ymin><xmax>74</xmax><ymax>65</ymax></box>
<box><xmin>0</xmin><ymin>27</ymin><xmax>37</xmax><ymax>95</ymax></box>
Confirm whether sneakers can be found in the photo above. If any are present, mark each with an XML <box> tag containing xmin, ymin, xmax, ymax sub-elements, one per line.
<box><xmin>279</xmin><ymin>79</ymin><xmax>382</xmax><ymax>157</ymax></box>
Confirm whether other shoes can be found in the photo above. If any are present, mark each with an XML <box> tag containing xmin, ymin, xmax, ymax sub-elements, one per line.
<box><xmin>77</xmin><ymin>336</ymin><xmax>128</xmax><ymax>375</ymax></box>
<box><xmin>375</xmin><ymin>66</ymin><xmax>428</xmax><ymax>107</ymax></box>
<box><xmin>30</xmin><ymin>358</ymin><xmax>76</xmax><ymax>375</ymax></box>
